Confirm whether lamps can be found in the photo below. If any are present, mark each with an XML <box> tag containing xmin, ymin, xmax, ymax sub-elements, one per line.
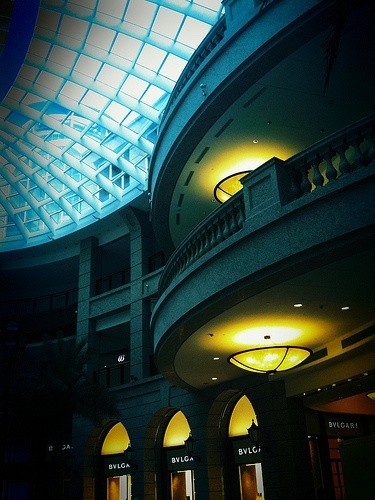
<box><xmin>226</xmin><ymin>334</ymin><xmax>314</xmax><ymax>379</ymax></box>
<box><xmin>214</xmin><ymin>170</ymin><xmax>254</xmax><ymax>205</ymax></box>
